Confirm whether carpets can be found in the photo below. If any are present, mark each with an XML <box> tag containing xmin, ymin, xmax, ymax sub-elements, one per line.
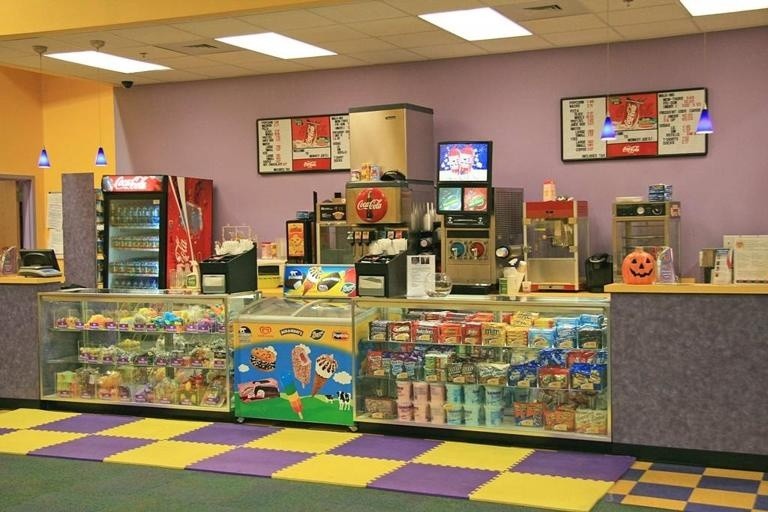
<box><xmin>0</xmin><ymin>405</ymin><xmax>767</xmax><ymax>512</ymax></box>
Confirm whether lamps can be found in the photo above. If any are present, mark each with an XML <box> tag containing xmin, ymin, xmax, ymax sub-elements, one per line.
<box><xmin>31</xmin><ymin>46</ymin><xmax>50</xmax><ymax>168</ymax></box>
<box><xmin>694</xmin><ymin>0</ymin><xmax>714</xmax><ymax>135</ymax></box>
<box><xmin>600</xmin><ymin>1</ymin><xmax>617</xmax><ymax>141</ymax></box>
<box><xmin>90</xmin><ymin>40</ymin><xmax>108</xmax><ymax>167</ymax></box>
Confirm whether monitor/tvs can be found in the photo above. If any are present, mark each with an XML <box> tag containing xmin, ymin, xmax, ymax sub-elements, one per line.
<box><xmin>19</xmin><ymin>249</ymin><xmax>59</xmax><ymax>271</ymax></box>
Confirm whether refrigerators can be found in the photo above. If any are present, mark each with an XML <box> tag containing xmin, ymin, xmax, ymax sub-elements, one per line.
<box><xmin>100</xmin><ymin>174</ymin><xmax>215</xmax><ymax>291</ymax></box>
<box><xmin>232</xmin><ymin>295</ymin><xmax>382</xmax><ymax>433</ymax></box>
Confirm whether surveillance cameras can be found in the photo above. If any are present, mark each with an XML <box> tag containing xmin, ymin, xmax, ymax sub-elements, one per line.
<box><xmin>121</xmin><ymin>80</ymin><xmax>133</xmax><ymax>88</ymax></box>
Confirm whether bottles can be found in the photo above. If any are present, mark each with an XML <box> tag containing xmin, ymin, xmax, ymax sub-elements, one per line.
<box><xmin>110</xmin><ymin>202</ymin><xmax>159</xmax><ymax>287</ymax></box>
<box><xmin>366</xmin><ymin>194</ymin><xmax>372</xmax><ymax>219</ymax></box>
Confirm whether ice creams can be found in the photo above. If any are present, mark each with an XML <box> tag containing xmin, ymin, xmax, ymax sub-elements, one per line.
<box><xmin>301</xmin><ymin>266</ymin><xmax>322</xmax><ymax>296</ymax></box>
<box><xmin>311</xmin><ymin>353</ymin><xmax>338</xmax><ymax>396</ymax></box>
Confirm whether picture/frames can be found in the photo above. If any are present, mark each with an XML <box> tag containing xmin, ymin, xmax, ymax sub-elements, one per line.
<box><xmin>255</xmin><ymin>113</ymin><xmax>349</xmax><ymax>175</ymax></box>
<box><xmin>560</xmin><ymin>87</ymin><xmax>708</xmax><ymax>164</ymax></box>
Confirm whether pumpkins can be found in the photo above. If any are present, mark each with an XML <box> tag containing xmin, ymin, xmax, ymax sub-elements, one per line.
<box><xmin>622</xmin><ymin>246</ymin><xmax>656</xmax><ymax>285</ymax></box>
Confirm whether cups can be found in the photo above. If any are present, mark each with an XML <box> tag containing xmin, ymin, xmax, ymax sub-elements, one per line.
<box><xmin>348</xmin><ymin>163</ymin><xmax>382</xmax><ymax>183</ymax></box>
<box><xmin>425</xmin><ymin>273</ymin><xmax>453</xmax><ymax>297</ymax></box>
<box><xmin>495</xmin><ymin>246</ymin><xmax>508</xmax><ymax>258</ymax></box>
<box><xmin>622</xmin><ymin>99</ymin><xmax>643</xmax><ymax>128</ymax></box>
<box><xmin>304</xmin><ymin>122</ymin><xmax>321</xmax><ymax>146</ymax></box>
<box><xmin>522</xmin><ymin>282</ymin><xmax>531</xmax><ymax>294</ymax></box>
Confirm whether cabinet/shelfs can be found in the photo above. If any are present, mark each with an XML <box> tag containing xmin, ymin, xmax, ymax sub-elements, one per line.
<box><xmin>38</xmin><ymin>291</ymin><xmax>262</xmax><ymax>414</ymax></box>
<box><xmin>351</xmin><ymin>291</ymin><xmax>611</xmax><ymax>441</ymax></box>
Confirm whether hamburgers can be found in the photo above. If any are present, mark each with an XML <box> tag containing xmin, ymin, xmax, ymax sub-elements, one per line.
<box><xmin>249</xmin><ymin>346</ymin><xmax>276</xmax><ymax>373</ymax></box>
<box><xmin>316</xmin><ymin>135</ymin><xmax>329</xmax><ymax>146</ymax></box>
<box><xmin>638</xmin><ymin>116</ymin><xmax>657</xmax><ymax>128</ymax></box>
